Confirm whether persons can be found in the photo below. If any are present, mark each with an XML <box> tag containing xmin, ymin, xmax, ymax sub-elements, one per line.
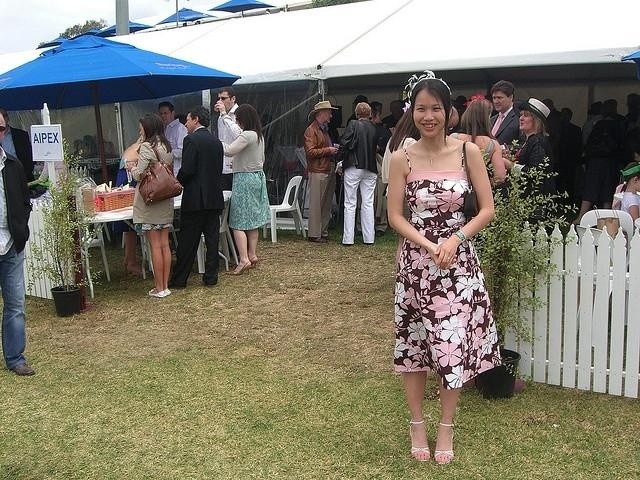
<box><xmin>114</xmin><ymin>85</ymin><xmax>271</xmax><ymax>299</ymax></box>
<box><xmin>337</xmin><ymin>78</ymin><xmax>640</xmax><ymax>306</ymax></box>
<box><xmin>304</xmin><ymin>102</ymin><xmax>339</xmax><ymax>243</ymax></box>
<box><xmin>386</xmin><ymin>75</ymin><xmax>494</xmax><ymax>465</ymax></box>
<box><xmin>0</xmin><ymin>106</ymin><xmax>34</xmax><ymax>184</ymax></box>
<box><xmin>0</xmin><ymin>144</ymin><xmax>35</xmax><ymax>376</ymax></box>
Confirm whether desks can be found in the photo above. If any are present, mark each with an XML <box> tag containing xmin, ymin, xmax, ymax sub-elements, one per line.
<box><xmin>83</xmin><ymin>190</ymin><xmax>232</xmax><ymax>279</ymax></box>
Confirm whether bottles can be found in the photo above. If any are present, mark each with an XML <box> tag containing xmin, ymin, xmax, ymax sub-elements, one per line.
<box><xmin>70</xmin><ymin>166</ymin><xmax>88</xmax><ymax>178</ymax></box>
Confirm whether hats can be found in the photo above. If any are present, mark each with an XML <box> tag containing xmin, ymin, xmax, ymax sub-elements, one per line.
<box><xmin>513</xmin><ymin>98</ymin><xmax>551</xmax><ymax>129</ymax></box>
<box><xmin>314</xmin><ymin>101</ymin><xmax>339</xmax><ymax>112</ymax></box>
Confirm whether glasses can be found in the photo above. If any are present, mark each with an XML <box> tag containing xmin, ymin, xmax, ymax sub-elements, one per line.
<box><xmin>216</xmin><ymin>97</ymin><xmax>230</xmax><ymax>100</ymax></box>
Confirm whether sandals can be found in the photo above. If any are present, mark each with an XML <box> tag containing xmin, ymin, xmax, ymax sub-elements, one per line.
<box><xmin>434</xmin><ymin>423</ymin><xmax>455</xmax><ymax>465</ymax></box>
<box><xmin>409</xmin><ymin>418</ymin><xmax>431</xmax><ymax>462</ymax></box>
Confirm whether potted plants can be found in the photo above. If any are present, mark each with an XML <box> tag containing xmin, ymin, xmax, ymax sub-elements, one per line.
<box><xmin>471</xmin><ymin>141</ymin><xmax>577</xmax><ymax>398</ymax></box>
<box><xmin>25</xmin><ymin>138</ymin><xmax>104</xmax><ymax>317</ymax></box>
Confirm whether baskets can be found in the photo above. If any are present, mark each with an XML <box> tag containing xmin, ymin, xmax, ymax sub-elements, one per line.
<box><xmin>95</xmin><ymin>188</ymin><xmax>135</xmax><ymax>212</ymax></box>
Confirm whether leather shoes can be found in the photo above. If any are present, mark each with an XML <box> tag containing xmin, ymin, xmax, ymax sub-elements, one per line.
<box><xmin>124</xmin><ymin>255</ymin><xmax>259</xmax><ymax>298</ymax></box>
<box><xmin>12</xmin><ymin>364</ymin><xmax>35</xmax><ymax>376</ymax></box>
<box><xmin>309</xmin><ymin>230</ymin><xmax>384</xmax><ymax>246</ymax></box>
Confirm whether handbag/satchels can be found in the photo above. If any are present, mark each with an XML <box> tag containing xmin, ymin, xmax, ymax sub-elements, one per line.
<box><xmin>597</xmin><ymin>218</ymin><xmax>619</xmax><ymax>240</ymax></box>
<box><xmin>139</xmin><ymin>162</ymin><xmax>182</xmax><ymax>205</ymax></box>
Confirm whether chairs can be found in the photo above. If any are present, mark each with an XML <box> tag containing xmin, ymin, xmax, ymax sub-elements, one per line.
<box><xmin>76</xmin><ymin>183</ymin><xmax>111</xmax><ymax>299</ymax></box>
<box><xmin>580</xmin><ymin>208</ymin><xmax>633</xmax><ymax>268</ymax></box>
<box><xmin>197</xmin><ymin>194</ymin><xmax>240</xmax><ymax>274</ymax></box>
<box><xmin>263</xmin><ymin>176</ymin><xmax>306</xmax><ymax>243</ymax></box>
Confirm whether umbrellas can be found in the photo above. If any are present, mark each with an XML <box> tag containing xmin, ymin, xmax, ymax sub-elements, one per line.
<box><xmin>0</xmin><ymin>36</ymin><xmax>240</xmax><ymax>186</ymax></box>
